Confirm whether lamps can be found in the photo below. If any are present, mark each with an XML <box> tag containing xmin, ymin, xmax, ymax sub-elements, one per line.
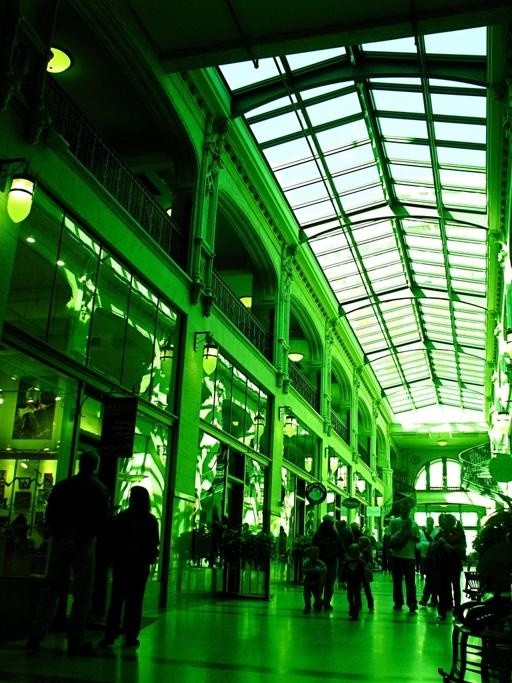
<box><xmin>304</xmin><ymin>455</ymin><xmax>314</xmax><ymax>473</ymax></box>
<box><xmin>284</xmin><ymin>414</ymin><xmax>298</xmax><ymax>439</ymax></box>
<box><xmin>358</xmin><ymin>477</ymin><xmax>366</xmax><ymax>494</ymax></box>
<box><xmin>7</xmin><ymin>157</ymin><xmax>35</xmax><ymax>224</ymax></box>
<box><xmin>330</xmin><ymin>451</ymin><xmax>339</xmax><ymax>473</ymax></box>
<box><xmin>376</xmin><ymin>493</ymin><xmax>384</xmax><ymax>507</ymax></box>
<box><xmin>203</xmin><ymin>342</ymin><xmax>219</xmax><ymax>375</ymax></box>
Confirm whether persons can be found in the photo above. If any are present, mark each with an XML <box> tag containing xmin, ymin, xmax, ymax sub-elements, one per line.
<box><xmin>95</xmin><ymin>486</ymin><xmax>160</xmax><ymax>652</ymax></box>
<box><xmin>24</xmin><ymin>450</ymin><xmax>111</xmax><ymax>658</ymax></box>
<box><xmin>381</xmin><ymin>506</ymin><xmax>467</xmax><ymax>621</ymax></box>
<box><xmin>301</xmin><ymin>514</ymin><xmax>375</xmax><ymax>620</ymax></box>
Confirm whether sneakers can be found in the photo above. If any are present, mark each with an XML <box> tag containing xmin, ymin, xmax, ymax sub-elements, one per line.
<box><xmin>24</xmin><ymin>636</ymin><xmax>138</xmax><ymax>658</ymax></box>
<box><xmin>394</xmin><ymin>599</ymin><xmax>458</xmax><ymax>621</ymax></box>
<box><xmin>350</xmin><ymin>604</ymin><xmax>373</xmax><ymax>619</ymax></box>
<box><xmin>305</xmin><ymin>600</ymin><xmax>333</xmax><ymax>613</ymax></box>
